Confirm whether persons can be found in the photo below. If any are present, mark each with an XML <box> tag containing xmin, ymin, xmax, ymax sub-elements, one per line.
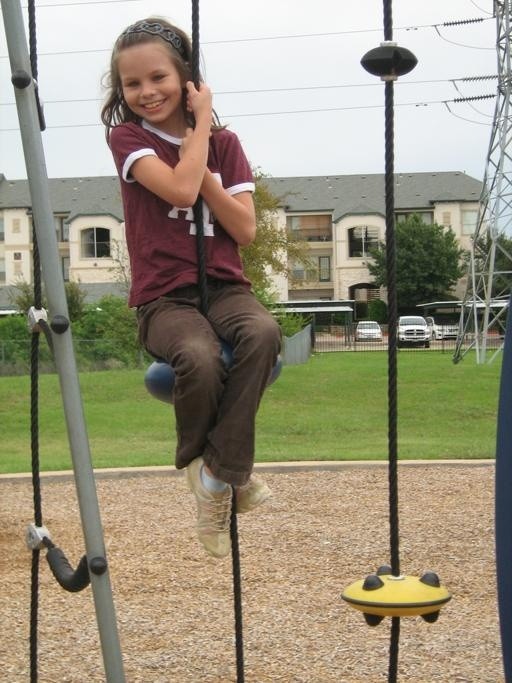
<box><xmin>100</xmin><ymin>17</ymin><xmax>283</xmax><ymax>560</ymax></box>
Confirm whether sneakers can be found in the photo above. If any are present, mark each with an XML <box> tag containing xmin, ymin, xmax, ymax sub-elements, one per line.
<box><xmin>188</xmin><ymin>457</ymin><xmax>233</xmax><ymax>558</ymax></box>
<box><xmin>235</xmin><ymin>475</ymin><xmax>271</xmax><ymax>514</ymax></box>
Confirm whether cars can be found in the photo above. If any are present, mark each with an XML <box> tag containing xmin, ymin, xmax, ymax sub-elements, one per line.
<box><xmin>355</xmin><ymin>320</ymin><xmax>383</xmax><ymax>342</ymax></box>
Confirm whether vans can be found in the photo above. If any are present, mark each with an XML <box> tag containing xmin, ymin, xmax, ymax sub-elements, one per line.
<box><xmin>424</xmin><ymin>316</ymin><xmax>460</xmax><ymax>341</ymax></box>
<box><xmin>396</xmin><ymin>315</ymin><xmax>431</xmax><ymax>348</ymax></box>
